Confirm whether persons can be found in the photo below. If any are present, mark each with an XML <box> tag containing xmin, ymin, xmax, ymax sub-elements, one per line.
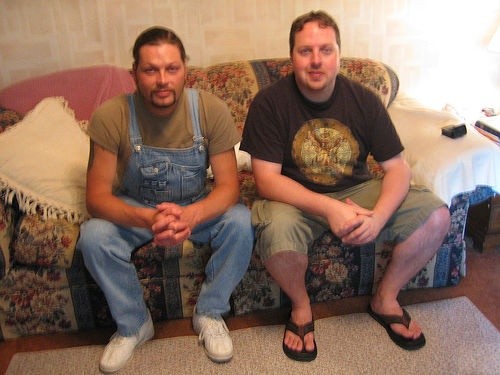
<box><xmin>239</xmin><ymin>11</ymin><xmax>450</xmax><ymax>362</ymax></box>
<box><xmin>75</xmin><ymin>26</ymin><xmax>254</xmax><ymax>373</ymax></box>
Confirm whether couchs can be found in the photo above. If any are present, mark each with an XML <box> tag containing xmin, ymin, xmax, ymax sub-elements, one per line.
<box><xmin>0</xmin><ymin>56</ymin><xmax>500</xmax><ymax>340</ymax></box>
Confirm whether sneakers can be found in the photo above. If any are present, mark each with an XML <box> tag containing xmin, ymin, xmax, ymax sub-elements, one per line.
<box><xmin>192</xmin><ymin>304</ymin><xmax>233</xmax><ymax>362</ymax></box>
<box><xmin>99</xmin><ymin>308</ymin><xmax>155</xmax><ymax>373</ymax></box>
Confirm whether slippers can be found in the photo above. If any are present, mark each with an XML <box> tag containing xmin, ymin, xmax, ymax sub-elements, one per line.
<box><xmin>282</xmin><ymin>317</ymin><xmax>317</xmax><ymax>362</ymax></box>
<box><xmin>367</xmin><ymin>302</ymin><xmax>426</xmax><ymax>350</ymax></box>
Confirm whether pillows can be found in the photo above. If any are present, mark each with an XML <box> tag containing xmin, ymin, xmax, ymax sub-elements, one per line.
<box><xmin>0</xmin><ymin>96</ymin><xmax>91</xmax><ymax>223</ymax></box>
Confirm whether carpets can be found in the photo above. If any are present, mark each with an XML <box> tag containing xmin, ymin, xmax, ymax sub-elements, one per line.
<box><xmin>6</xmin><ymin>296</ymin><xmax>500</xmax><ymax>375</ymax></box>
